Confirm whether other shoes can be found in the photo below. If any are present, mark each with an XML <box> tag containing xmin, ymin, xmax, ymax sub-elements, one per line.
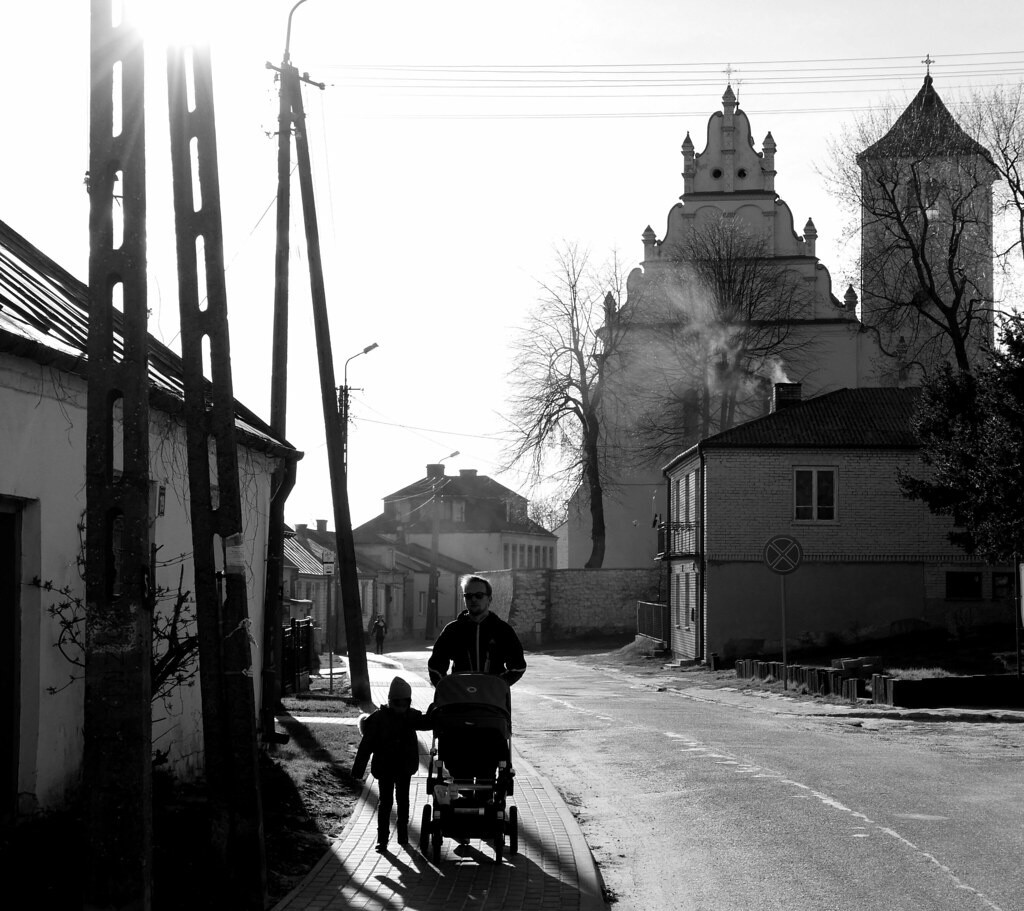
<box><xmin>376</xmin><ymin>842</ymin><xmax>387</xmax><ymax>849</ymax></box>
<box><xmin>398</xmin><ymin>837</ymin><xmax>408</xmax><ymax>845</ymax></box>
<box><xmin>453</xmin><ymin>835</ymin><xmax>470</xmax><ymax>845</ymax></box>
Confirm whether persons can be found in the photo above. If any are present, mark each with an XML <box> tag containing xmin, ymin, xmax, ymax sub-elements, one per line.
<box><xmin>350</xmin><ymin>676</ymin><xmax>437</xmax><ymax>849</ymax></box>
<box><xmin>428</xmin><ymin>577</ymin><xmax>527</xmax><ymax>844</ymax></box>
<box><xmin>370</xmin><ymin>616</ymin><xmax>387</xmax><ymax>655</ymax></box>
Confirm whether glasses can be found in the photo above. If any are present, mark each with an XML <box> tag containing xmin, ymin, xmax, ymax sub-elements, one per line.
<box><xmin>463</xmin><ymin>591</ymin><xmax>490</xmax><ymax>600</ymax></box>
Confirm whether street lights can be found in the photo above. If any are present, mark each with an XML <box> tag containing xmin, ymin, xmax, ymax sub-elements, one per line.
<box><xmin>334</xmin><ymin>342</ymin><xmax>379</xmax><ymax>655</ymax></box>
<box><xmin>425</xmin><ymin>451</ymin><xmax>460</xmax><ymax>638</ymax></box>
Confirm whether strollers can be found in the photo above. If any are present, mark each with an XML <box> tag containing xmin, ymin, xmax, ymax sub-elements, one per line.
<box><xmin>420</xmin><ymin>675</ymin><xmax>518</xmax><ymax>861</ymax></box>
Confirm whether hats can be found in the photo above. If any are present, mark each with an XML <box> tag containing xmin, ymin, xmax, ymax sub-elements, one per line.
<box><xmin>388</xmin><ymin>677</ymin><xmax>411</xmax><ymax>700</ymax></box>
